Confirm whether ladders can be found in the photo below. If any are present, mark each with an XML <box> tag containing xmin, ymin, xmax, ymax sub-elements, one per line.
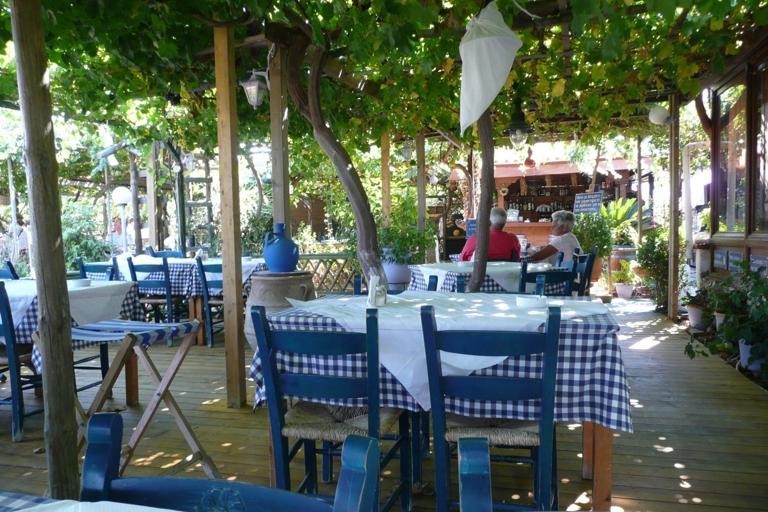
<box><xmin>182</xmin><ymin>145</ymin><xmax>217</xmax><ymax>257</ymax></box>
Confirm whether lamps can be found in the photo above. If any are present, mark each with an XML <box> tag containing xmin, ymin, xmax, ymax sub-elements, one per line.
<box><xmin>646</xmin><ymin>104</ymin><xmax>676</xmax><ymax>128</ymax></box>
<box><xmin>237</xmin><ymin>65</ymin><xmax>273</xmax><ymax>112</ymax></box>
<box><xmin>500</xmin><ymin>97</ymin><xmax>537</xmax><ymax>152</ymax></box>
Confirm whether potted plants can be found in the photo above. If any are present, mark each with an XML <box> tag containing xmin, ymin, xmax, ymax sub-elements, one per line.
<box><xmin>572</xmin><ymin>211</ymin><xmax>614</xmax><ymax>283</ymax></box>
<box><xmin>677</xmin><ymin>254</ymin><xmax>768</xmax><ymax>381</ymax></box>
<box><xmin>604</xmin><ymin>224</ymin><xmax>641</xmax><ymax>288</ymax></box>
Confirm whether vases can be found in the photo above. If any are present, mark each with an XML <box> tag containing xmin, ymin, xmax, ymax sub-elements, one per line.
<box><xmin>259</xmin><ymin>221</ymin><xmax>302</xmax><ymax>273</ymax></box>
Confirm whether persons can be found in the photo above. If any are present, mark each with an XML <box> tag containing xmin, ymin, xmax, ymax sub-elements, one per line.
<box><xmin>520</xmin><ymin>210</ymin><xmax>590</xmax><ymax>295</ymax></box>
<box><xmin>445</xmin><ymin>212</ymin><xmax>467</xmax><ymax>264</ymax></box>
<box><xmin>460</xmin><ymin>208</ymin><xmax>521</xmax><ymax>263</ymax></box>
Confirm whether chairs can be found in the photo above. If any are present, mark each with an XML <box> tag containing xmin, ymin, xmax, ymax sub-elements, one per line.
<box><xmin>0</xmin><ymin>234</ymin><xmax>634</xmax><ymax>512</ymax></box>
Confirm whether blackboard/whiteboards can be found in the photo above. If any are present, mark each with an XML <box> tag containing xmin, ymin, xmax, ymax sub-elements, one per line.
<box><xmin>572</xmin><ymin>191</ymin><xmax>603</xmax><ymax>215</ymax></box>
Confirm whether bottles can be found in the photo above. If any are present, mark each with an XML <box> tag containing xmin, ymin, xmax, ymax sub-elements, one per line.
<box><xmin>509</xmin><ymin>185</ymin><xmax>575</xmax><ymax>211</ymax></box>
<box><xmin>186</xmin><ymin>249</ymin><xmax>209</xmax><ymax>261</ymax></box>
<box><xmin>366</xmin><ymin>274</ymin><xmax>388</xmax><ymax>307</ymax></box>
<box><xmin>263</xmin><ymin>222</ymin><xmax>297</xmax><ymax>271</ymax></box>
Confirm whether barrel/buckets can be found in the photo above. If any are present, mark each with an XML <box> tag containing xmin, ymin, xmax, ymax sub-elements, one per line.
<box><xmin>616</xmin><ymin>283</ymin><xmax>633</xmax><ymax>298</ymax></box>
<box><xmin>466</xmin><ymin>218</ymin><xmax>477</xmax><ymax>238</ymax></box>
<box><xmin>611</xmin><ymin>248</ymin><xmax>636</xmax><ymax>279</ymax></box>
